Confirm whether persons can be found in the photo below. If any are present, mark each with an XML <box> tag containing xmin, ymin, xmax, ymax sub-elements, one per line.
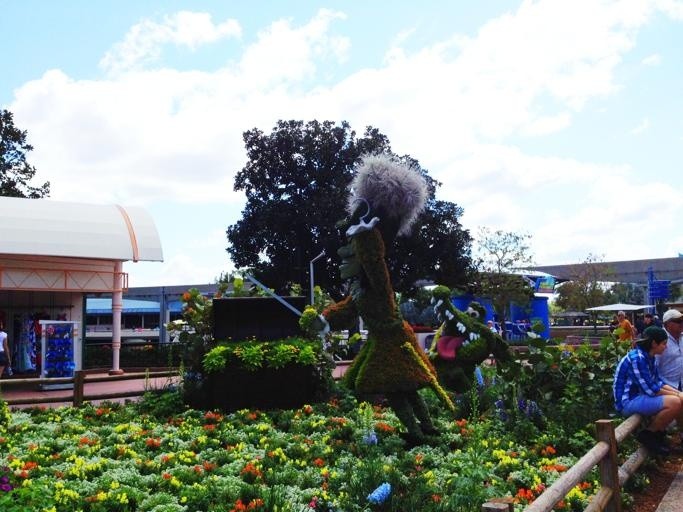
<box><xmin>0</xmin><ymin>319</ymin><xmax>11</xmax><ymax>378</ymax></box>
<box><xmin>610</xmin><ymin>308</ymin><xmax>682</xmax><ymax>451</ymax></box>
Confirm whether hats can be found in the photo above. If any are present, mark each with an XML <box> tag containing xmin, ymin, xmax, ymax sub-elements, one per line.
<box><xmin>661</xmin><ymin>309</ymin><xmax>683</xmax><ymax>323</ymax></box>
<box><xmin>618</xmin><ymin>311</ymin><xmax>625</xmax><ymax>317</ymax></box>
<box><xmin>636</xmin><ymin>325</ymin><xmax>668</xmax><ymax>343</ymax></box>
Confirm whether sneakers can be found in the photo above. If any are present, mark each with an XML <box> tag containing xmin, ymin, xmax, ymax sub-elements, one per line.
<box><xmin>636</xmin><ymin>429</ymin><xmax>670</xmax><ymax>455</ymax></box>
